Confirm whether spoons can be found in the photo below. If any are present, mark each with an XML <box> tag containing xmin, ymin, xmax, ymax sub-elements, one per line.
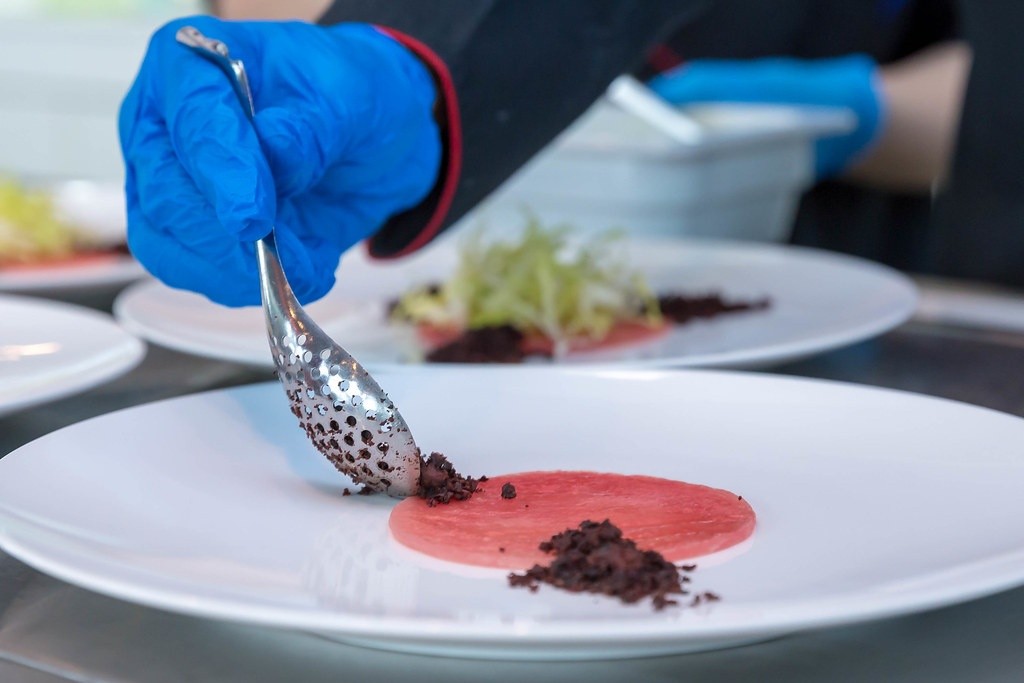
<box><xmin>174</xmin><ymin>24</ymin><xmax>422</xmax><ymax>500</ymax></box>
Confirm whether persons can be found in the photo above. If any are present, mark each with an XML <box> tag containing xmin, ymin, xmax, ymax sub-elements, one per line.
<box><xmin>118</xmin><ymin>0</ymin><xmax>1024</xmax><ymax>307</ymax></box>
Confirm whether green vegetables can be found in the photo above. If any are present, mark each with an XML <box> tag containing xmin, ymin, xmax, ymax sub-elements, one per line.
<box><xmin>404</xmin><ymin>221</ymin><xmax>660</xmax><ymax>355</ymax></box>
<box><xmin>0</xmin><ymin>181</ymin><xmax>73</xmax><ymax>261</ymax></box>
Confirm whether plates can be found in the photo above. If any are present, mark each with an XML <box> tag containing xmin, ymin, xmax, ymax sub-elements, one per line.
<box><xmin>0</xmin><ymin>290</ymin><xmax>147</xmax><ymax>422</ymax></box>
<box><xmin>0</xmin><ymin>178</ymin><xmax>148</xmax><ymax>306</ymax></box>
<box><xmin>113</xmin><ymin>234</ymin><xmax>922</xmax><ymax>372</ymax></box>
<box><xmin>0</xmin><ymin>366</ymin><xmax>1024</xmax><ymax>665</ymax></box>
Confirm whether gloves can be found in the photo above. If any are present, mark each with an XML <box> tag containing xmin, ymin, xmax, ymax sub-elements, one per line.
<box><xmin>646</xmin><ymin>53</ymin><xmax>885</xmax><ymax>176</ymax></box>
<box><xmin>114</xmin><ymin>15</ymin><xmax>441</xmax><ymax>310</ymax></box>
